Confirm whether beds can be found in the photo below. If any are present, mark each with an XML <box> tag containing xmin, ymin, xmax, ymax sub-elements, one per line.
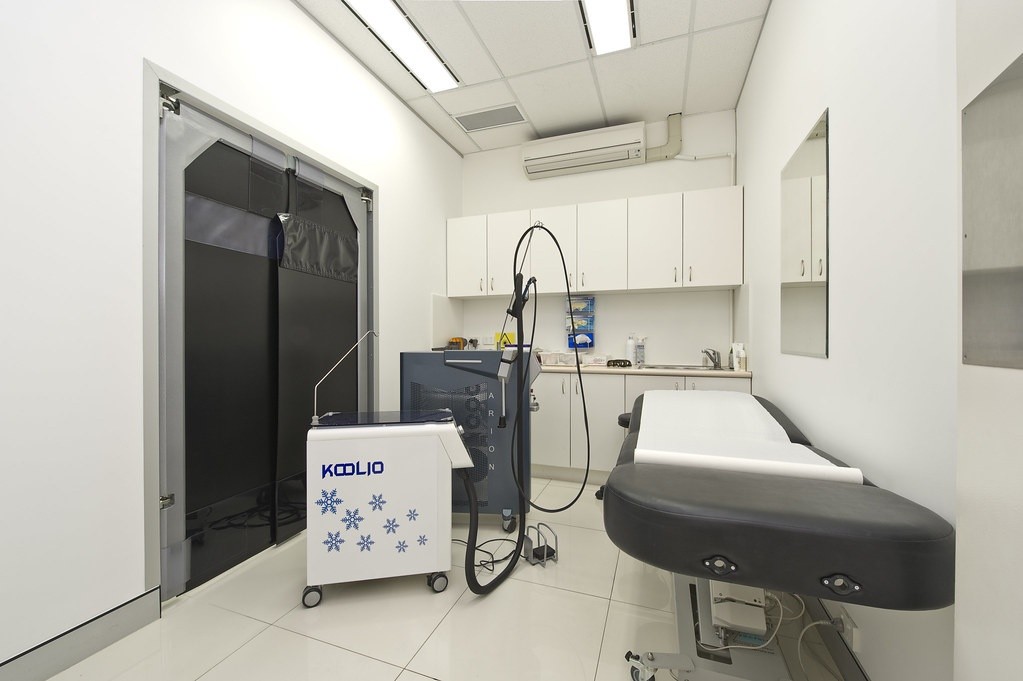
<box><xmin>594</xmin><ymin>389</ymin><xmax>956</xmax><ymax>681</ymax></box>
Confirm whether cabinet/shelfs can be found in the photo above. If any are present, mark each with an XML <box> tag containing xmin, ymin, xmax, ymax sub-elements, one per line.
<box><xmin>529</xmin><ymin>366</ymin><xmax>625</xmax><ymax>484</ymax></box>
<box><xmin>782</xmin><ymin>174</ymin><xmax>827</xmax><ymax>289</ymax></box>
<box><xmin>628</xmin><ymin>184</ymin><xmax>744</xmax><ymax>294</ymax></box>
<box><xmin>624</xmin><ymin>364</ymin><xmax>752</xmax><ymax>438</ymax></box>
<box><xmin>446</xmin><ymin>209</ymin><xmax>531</xmax><ymax>301</ymax></box>
<box><xmin>531</xmin><ymin>198</ymin><xmax>628</xmax><ymax>298</ymax></box>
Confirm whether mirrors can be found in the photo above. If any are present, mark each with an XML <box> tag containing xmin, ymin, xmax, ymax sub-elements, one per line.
<box><xmin>961</xmin><ymin>53</ymin><xmax>1023</xmax><ymax>369</ymax></box>
<box><xmin>781</xmin><ymin>107</ymin><xmax>829</xmax><ymax>359</ymax></box>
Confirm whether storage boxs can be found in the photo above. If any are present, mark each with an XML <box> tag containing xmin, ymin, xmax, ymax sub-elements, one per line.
<box><xmin>538</xmin><ymin>351</ymin><xmax>588</xmax><ymax>367</ymax></box>
<box><xmin>583</xmin><ymin>355</ymin><xmax>611</xmax><ymax>366</ymax></box>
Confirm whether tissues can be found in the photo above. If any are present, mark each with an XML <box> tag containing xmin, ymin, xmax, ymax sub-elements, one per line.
<box><xmin>567</xmin><ymin>332</ymin><xmax>594</xmax><ymax>349</ymax></box>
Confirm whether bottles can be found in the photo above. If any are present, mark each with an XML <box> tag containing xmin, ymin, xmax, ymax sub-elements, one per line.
<box><xmin>737</xmin><ymin>350</ymin><xmax>746</xmax><ymax>371</ymax></box>
<box><xmin>627</xmin><ymin>336</ymin><xmax>636</xmax><ymax>365</ymax></box>
<box><xmin>636</xmin><ymin>339</ymin><xmax>644</xmax><ymax>364</ymax></box>
<box><xmin>729</xmin><ymin>348</ymin><xmax>733</xmax><ymax>369</ymax></box>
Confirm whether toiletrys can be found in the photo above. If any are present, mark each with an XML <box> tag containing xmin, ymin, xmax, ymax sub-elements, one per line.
<box><xmin>728</xmin><ymin>342</ymin><xmax>747</xmax><ymax>372</ymax></box>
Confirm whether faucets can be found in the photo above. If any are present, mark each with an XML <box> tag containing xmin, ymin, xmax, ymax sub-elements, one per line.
<box><xmin>701</xmin><ymin>347</ymin><xmax>723</xmax><ymax>370</ymax></box>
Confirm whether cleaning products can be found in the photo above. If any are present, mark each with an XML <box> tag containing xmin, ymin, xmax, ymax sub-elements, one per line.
<box><xmin>626</xmin><ymin>335</ymin><xmax>648</xmax><ymax>365</ymax></box>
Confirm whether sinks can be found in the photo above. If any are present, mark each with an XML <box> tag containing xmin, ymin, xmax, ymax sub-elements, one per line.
<box><xmin>637</xmin><ymin>363</ymin><xmax>725</xmax><ymax>371</ymax></box>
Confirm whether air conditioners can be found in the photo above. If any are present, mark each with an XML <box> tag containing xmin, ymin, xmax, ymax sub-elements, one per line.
<box><xmin>522</xmin><ymin>120</ymin><xmax>647</xmax><ymax>180</ymax></box>
